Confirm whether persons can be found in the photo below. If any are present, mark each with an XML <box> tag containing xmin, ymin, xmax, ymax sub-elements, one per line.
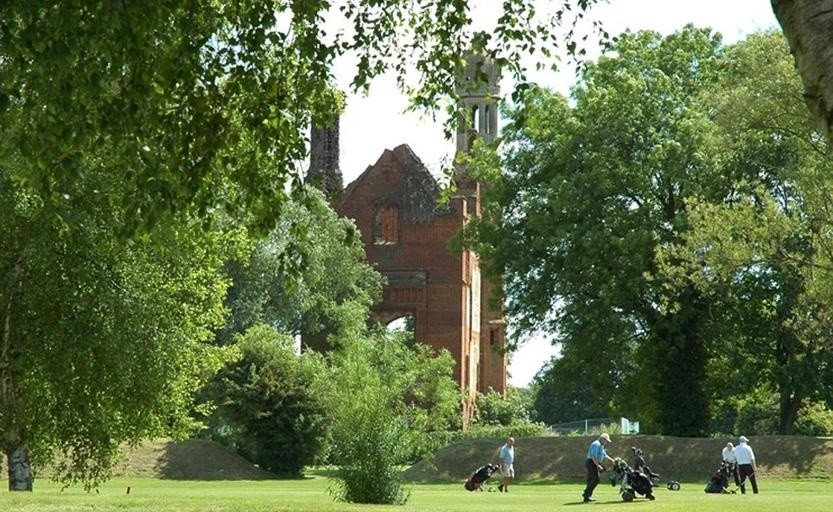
<box><xmin>582</xmin><ymin>433</ymin><xmax>614</xmax><ymax>501</ymax></box>
<box><xmin>635</xmin><ymin>463</ymin><xmax>646</xmax><ymax>474</ymax></box>
<box><xmin>722</xmin><ymin>442</ymin><xmax>739</xmax><ymax>486</ymax></box>
<box><xmin>498</xmin><ymin>438</ymin><xmax>514</xmax><ymax>492</ymax></box>
<box><xmin>732</xmin><ymin>436</ymin><xmax>758</xmax><ymax>494</ymax></box>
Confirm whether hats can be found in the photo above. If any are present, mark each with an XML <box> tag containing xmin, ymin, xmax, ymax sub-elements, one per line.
<box><xmin>601</xmin><ymin>432</ymin><xmax>612</xmax><ymax>443</ymax></box>
<box><xmin>739</xmin><ymin>436</ymin><xmax>749</xmax><ymax>441</ymax></box>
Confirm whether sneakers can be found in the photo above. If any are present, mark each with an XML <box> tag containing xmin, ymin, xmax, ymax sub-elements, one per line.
<box><xmin>582</xmin><ymin>493</ymin><xmax>596</xmax><ymax>502</ymax></box>
<box><xmin>498</xmin><ymin>485</ymin><xmax>502</xmax><ymax>492</ymax></box>
